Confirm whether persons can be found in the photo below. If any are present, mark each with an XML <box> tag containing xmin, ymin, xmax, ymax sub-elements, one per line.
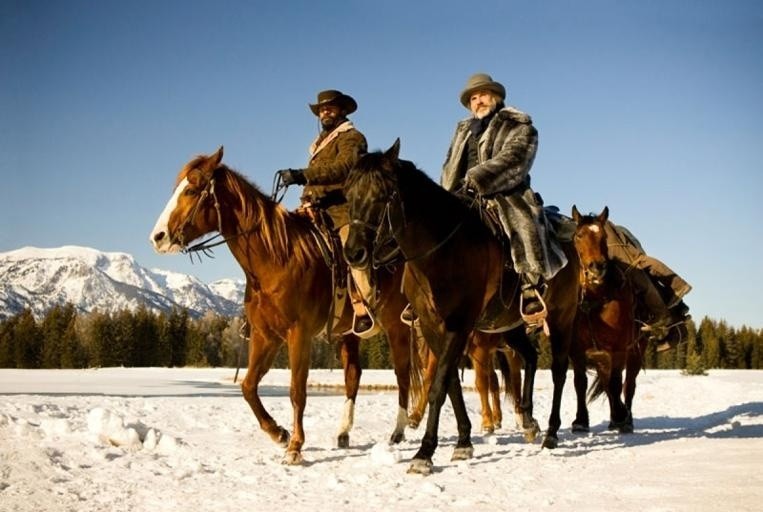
<box><xmin>401</xmin><ymin>73</ymin><xmax>568</xmax><ymax>335</ymax></box>
<box><xmin>607</xmin><ymin>220</ymin><xmax>694</xmax><ymax>353</ymax></box>
<box><xmin>280</xmin><ymin>90</ymin><xmax>374</xmax><ymax>333</ymax></box>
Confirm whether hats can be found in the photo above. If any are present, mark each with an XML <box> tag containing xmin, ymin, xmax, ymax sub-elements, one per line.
<box><xmin>308</xmin><ymin>90</ymin><xmax>356</xmax><ymax>115</ymax></box>
<box><xmin>458</xmin><ymin>74</ymin><xmax>505</xmax><ymax>108</ymax></box>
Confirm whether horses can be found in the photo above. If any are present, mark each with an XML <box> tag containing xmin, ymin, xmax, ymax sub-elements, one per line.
<box><xmin>343</xmin><ymin>136</ymin><xmax>582</xmax><ymax>474</ymax></box>
<box><xmin>570</xmin><ymin>204</ymin><xmax>648</xmax><ymax>436</ymax></box>
<box><xmin>149</xmin><ymin>145</ymin><xmax>424</xmax><ymax>466</ymax></box>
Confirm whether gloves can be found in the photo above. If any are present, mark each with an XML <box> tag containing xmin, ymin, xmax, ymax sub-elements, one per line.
<box><xmin>279</xmin><ymin>169</ymin><xmax>306</xmax><ymax>186</ymax></box>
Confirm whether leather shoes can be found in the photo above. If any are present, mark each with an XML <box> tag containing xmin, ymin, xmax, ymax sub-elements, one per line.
<box><xmin>355</xmin><ymin>317</ymin><xmax>371</xmax><ymax>331</ymax></box>
<box><xmin>402</xmin><ymin>308</ymin><xmax>416</xmax><ymax>320</ymax></box>
<box><xmin>523</xmin><ymin>279</ymin><xmax>548</xmax><ymax>313</ymax></box>
